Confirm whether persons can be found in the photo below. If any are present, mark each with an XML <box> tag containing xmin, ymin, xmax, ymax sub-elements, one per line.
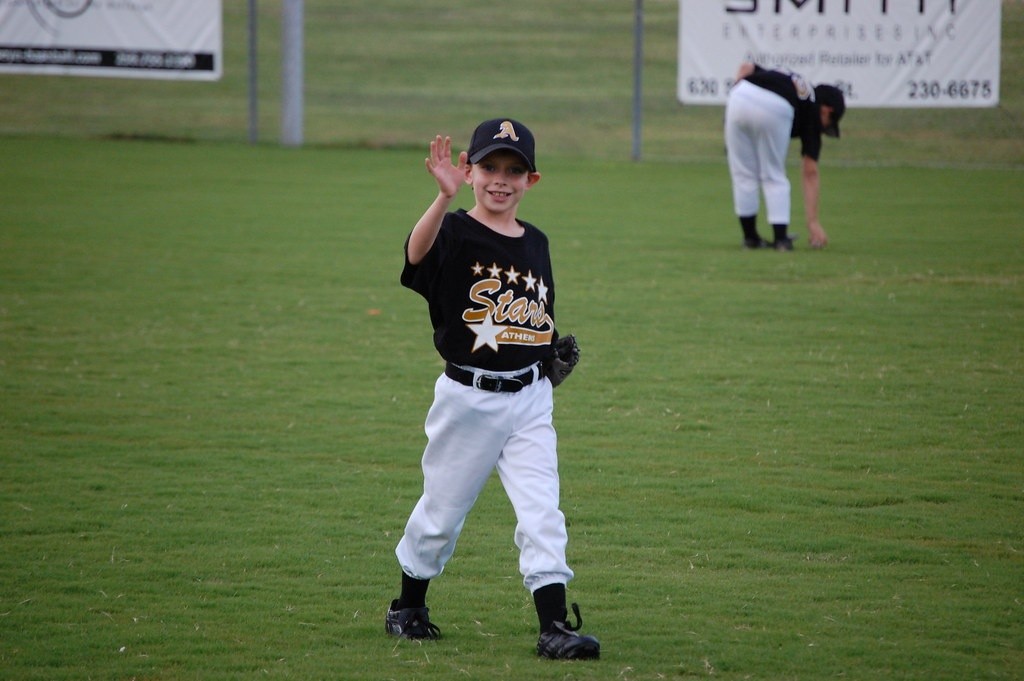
<box><xmin>725</xmin><ymin>63</ymin><xmax>845</xmax><ymax>250</ymax></box>
<box><xmin>385</xmin><ymin>118</ymin><xmax>601</xmax><ymax>659</ymax></box>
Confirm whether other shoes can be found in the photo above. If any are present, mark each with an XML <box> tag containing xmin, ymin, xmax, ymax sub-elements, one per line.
<box><xmin>743</xmin><ymin>236</ymin><xmax>772</xmax><ymax>250</ymax></box>
<box><xmin>775</xmin><ymin>239</ymin><xmax>792</xmax><ymax>251</ymax></box>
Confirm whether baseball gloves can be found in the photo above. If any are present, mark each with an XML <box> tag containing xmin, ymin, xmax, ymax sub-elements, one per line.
<box><xmin>547</xmin><ymin>332</ymin><xmax>581</xmax><ymax>389</ymax></box>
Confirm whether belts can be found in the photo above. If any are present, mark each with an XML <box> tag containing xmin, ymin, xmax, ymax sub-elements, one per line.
<box><xmin>442</xmin><ymin>363</ymin><xmax>546</xmax><ymax>393</ymax></box>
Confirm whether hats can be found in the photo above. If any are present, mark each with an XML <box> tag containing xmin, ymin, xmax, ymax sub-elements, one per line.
<box><xmin>466</xmin><ymin>117</ymin><xmax>536</xmax><ymax>173</ymax></box>
<box><xmin>816</xmin><ymin>84</ymin><xmax>847</xmax><ymax>138</ymax></box>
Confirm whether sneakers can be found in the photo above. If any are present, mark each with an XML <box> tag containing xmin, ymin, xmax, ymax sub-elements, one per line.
<box><xmin>536</xmin><ymin>603</ymin><xmax>601</xmax><ymax>661</ymax></box>
<box><xmin>384</xmin><ymin>598</ymin><xmax>441</xmax><ymax>642</ymax></box>
